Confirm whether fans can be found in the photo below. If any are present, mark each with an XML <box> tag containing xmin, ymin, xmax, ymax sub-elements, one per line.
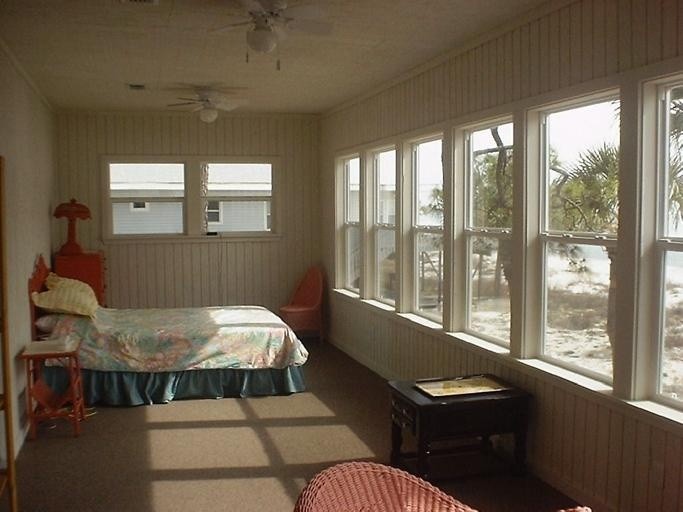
<box><xmin>164</xmin><ymin>87</ymin><xmax>241</xmax><ymax>114</ymax></box>
<box><xmin>203</xmin><ymin>0</ymin><xmax>333</xmax><ymax>40</ymax></box>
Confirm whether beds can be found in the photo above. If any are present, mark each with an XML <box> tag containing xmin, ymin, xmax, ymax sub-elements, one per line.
<box><xmin>27</xmin><ymin>253</ymin><xmax>294</xmax><ymax>407</ymax></box>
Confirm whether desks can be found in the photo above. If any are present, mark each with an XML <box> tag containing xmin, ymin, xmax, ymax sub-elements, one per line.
<box><xmin>16</xmin><ymin>338</ymin><xmax>92</xmax><ymax>441</ymax></box>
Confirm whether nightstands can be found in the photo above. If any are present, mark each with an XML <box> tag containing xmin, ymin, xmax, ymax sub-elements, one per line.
<box><xmin>53</xmin><ymin>250</ymin><xmax>106</xmax><ymax>307</ymax></box>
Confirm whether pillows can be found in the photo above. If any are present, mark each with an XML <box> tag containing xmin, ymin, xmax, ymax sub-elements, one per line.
<box><xmin>31</xmin><ymin>272</ymin><xmax>98</xmax><ymax>335</ymax></box>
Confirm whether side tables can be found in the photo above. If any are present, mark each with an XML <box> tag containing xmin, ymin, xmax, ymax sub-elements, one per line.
<box><xmin>386</xmin><ymin>373</ymin><xmax>532</xmax><ymax>486</ymax></box>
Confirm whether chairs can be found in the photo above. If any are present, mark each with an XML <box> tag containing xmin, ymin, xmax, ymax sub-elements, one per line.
<box><xmin>291</xmin><ymin>461</ymin><xmax>591</xmax><ymax>512</ymax></box>
<box><xmin>278</xmin><ymin>264</ymin><xmax>325</xmax><ymax>347</ymax></box>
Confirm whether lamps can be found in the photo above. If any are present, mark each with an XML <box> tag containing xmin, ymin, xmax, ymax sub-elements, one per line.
<box><xmin>200</xmin><ymin>104</ymin><xmax>217</xmax><ymax>123</ymax></box>
<box><xmin>53</xmin><ymin>198</ymin><xmax>92</xmax><ymax>255</ymax></box>
<box><xmin>244</xmin><ymin>17</ymin><xmax>279</xmax><ymax>57</ymax></box>
